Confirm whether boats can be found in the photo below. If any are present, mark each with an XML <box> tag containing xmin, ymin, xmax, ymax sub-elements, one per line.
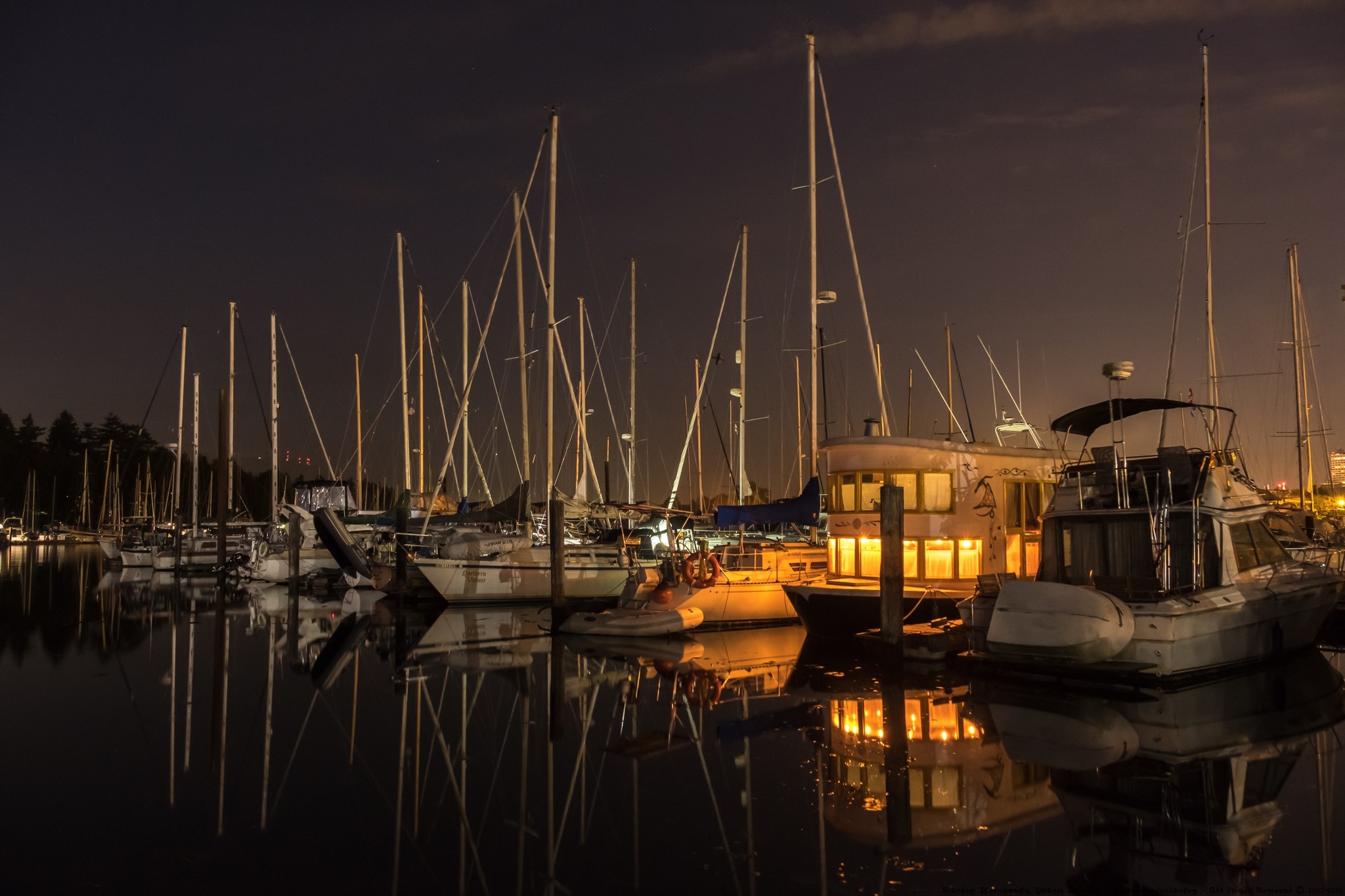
<box><xmin>956</xmin><ymin>398</ymin><xmax>1345</xmax><ymax>689</ymax></box>
<box><xmin>393</xmin><ymin>522</ymin><xmax>684</xmax><ymax>608</ymax></box>
<box><xmin>1256</xmin><ymin>479</ymin><xmax>1345</xmax><ymax>572</ymax></box>
<box><xmin>559</xmin><ymin>525</ymin><xmax>830</xmax><ymax>636</ymax></box>
<box><xmin>782</xmin><ymin>418</ymin><xmax>1094</xmax><ymax>667</ymax></box>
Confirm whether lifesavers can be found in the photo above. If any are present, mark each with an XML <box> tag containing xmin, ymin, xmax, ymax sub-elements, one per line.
<box><xmin>683</xmin><ymin>551</ymin><xmax>720</xmax><ymax>589</ymax></box>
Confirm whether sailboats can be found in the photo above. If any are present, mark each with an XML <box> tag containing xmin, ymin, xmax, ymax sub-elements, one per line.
<box><xmin>94</xmin><ymin>562</ymin><xmax>1344</xmax><ymax>883</ymax></box>
<box><xmin>410</xmin><ymin>39</ymin><xmax>890</xmax><ymax>552</ymax></box>
<box><xmin>2</xmin><ymin>229</ymin><xmax>420</xmax><ymax>589</ymax></box>
